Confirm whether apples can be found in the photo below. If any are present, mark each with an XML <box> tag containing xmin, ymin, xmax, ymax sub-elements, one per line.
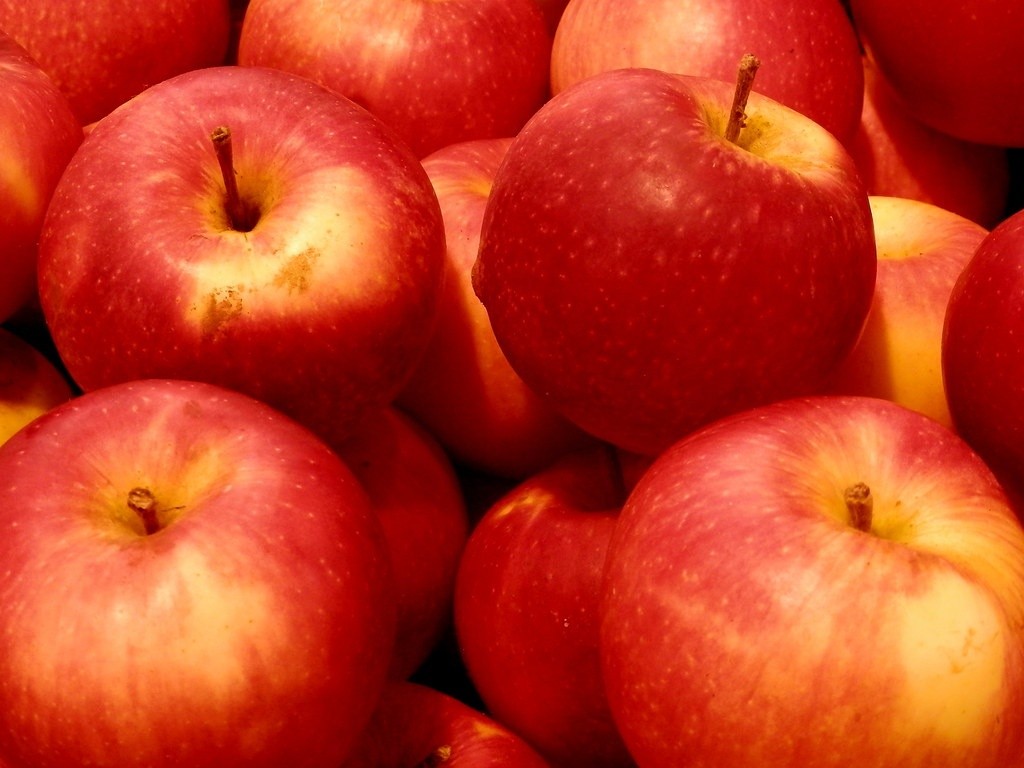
<box><xmin>0</xmin><ymin>0</ymin><xmax>1024</xmax><ymax>768</ymax></box>
